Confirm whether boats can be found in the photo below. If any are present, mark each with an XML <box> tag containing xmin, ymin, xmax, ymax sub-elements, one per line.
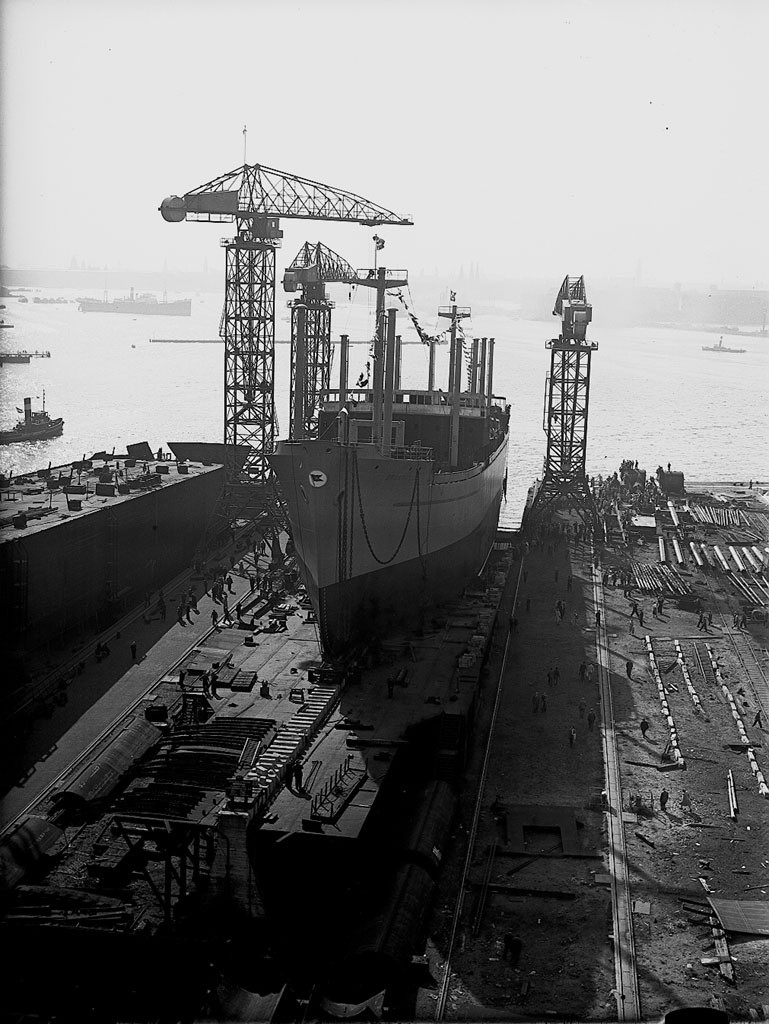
<box><xmin>0</xmin><ymin>352</ymin><xmax>32</xmax><ymax>363</ymax></box>
<box><xmin>0</xmin><ymin>388</ymin><xmax>65</xmax><ymax>445</ymax></box>
<box><xmin>18</xmin><ymin>297</ymin><xmax>28</xmax><ymax>303</ymax></box>
<box><xmin>76</xmin><ymin>285</ymin><xmax>192</xmax><ymax>316</ymax></box>
<box><xmin>76</xmin><ymin>296</ymin><xmax>102</xmax><ymax>303</ymax></box>
<box><xmin>33</xmin><ymin>296</ymin><xmax>68</xmax><ymax>303</ymax></box>
<box><xmin>702</xmin><ymin>336</ymin><xmax>746</xmax><ymax>353</ymax></box>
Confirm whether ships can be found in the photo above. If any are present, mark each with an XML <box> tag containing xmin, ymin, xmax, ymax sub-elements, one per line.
<box><xmin>263</xmin><ymin>233</ymin><xmax>511</xmax><ymax>657</ymax></box>
<box><xmin>0</xmin><ymin>440</ymin><xmax>251</xmax><ymax>686</ymax></box>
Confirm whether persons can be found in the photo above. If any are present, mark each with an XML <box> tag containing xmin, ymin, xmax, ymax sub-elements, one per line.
<box><xmin>192</xmin><ymin>558</ymin><xmax>233</xmax><ymax>601</ymax></box>
<box><xmin>177</xmin><ymin>589</ymin><xmax>197</xmax><ymax>619</ymax></box>
<box><xmin>586</xmin><ymin>460</ymin><xmax>670</xmax><ymax>487</ymax></box>
<box><xmin>640</xmin><ymin>719</ymin><xmax>648</xmax><ymax>738</ymax></box>
<box><xmin>179</xmin><ymin>670</ymin><xmax>185</xmax><ymax>688</ymax></box>
<box><xmin>246</xmin><ymin>538</ymin><xmax>266</xmax><ymax>565</ymax></box>
<box><xmin>749</xmin><ymin>479</ymin><xmax>753</xmax><ymax>489</ymax></box>
<box><xmin>144</xmin><ymin>589</ymin><xmax>166</xmax><ymax>619</ymax></box>
<box><xmin>532</xmin><ymin>660</ymin><xmax>596</xmax><ymax>746</ymax></box>
<box><xmin>659</xmin><ymin>788</ymin><xmax>668</xmax><ymax>812</ymax></box>
<box><xmin>230</xmin><ymin>555</ymin><xmax>245</xmax><ymax>576</ymax></box>
<box><xmin>210</xmin><ymin>595</ymin><xmax>242</xmax><ymax>625</ymax></box>
<box><xmin>131</xmin><ymin>642</ymin><xmax>137</xmax><ymax>659</ymax></box>
<box><xmin>752</xmin><ymin>710</ymin><xmax>763</xmax><ymax>728</ymax></box>
<box><xmin>199</xmin><ymin>671</ymin><xmax>217</xmax><ymax>694</ymax></box>
<box><xmin>679</xmin><ymin>790</ymin><xmax>693</xmax><ymax>812</ymax></box>
<box><xmin>387</xmin><ymin>678</ymin><xmax>395</xmax><ymax>698</ymax></box>
<box><xmin>626</xmin><ymin>660</ymin><xmax>633</xmax><ymax>678</ymax></box>
<box><xmin>593</xmin><ymin>556</ymin><xmax>665</xmax><ymax>633</ymax></box>
<box><xmin>96</xmin><ymin>642</ymin><xmax>109</xmax><ymax>663</ymax></box>
<box><xmin>536</xmin><ymin>508</ymin><xmax>594</xmax><ymax>559</ymax></box>
<box><xmin>508</xmin><ymin>568</ymin><xmax>578</xmax><ymax>634</ymax></box>
<box><xmin>489</xmin><ymin>793</ymin><xmax>512</xmax><ymax>846</ymax></box>
<box><xmin>285</xmin><ymin>759</ymin><xmax>304</xmax><ymax>789</ymax></box>
<box><xmin>731</xmin><ymin>606</ymin><xmax>752</xmax><ymax>631</ymax></box>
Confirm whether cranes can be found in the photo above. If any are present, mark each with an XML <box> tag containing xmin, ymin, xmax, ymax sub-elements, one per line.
<box><xmin>523</xmin><ymin>274</ymin><xmax>608</xmax><ymax>543</ymax></box>
<box><xmin>280</xmin><ymin>241</ymin><xmax>360</xmax><ymax>441</ymax></box>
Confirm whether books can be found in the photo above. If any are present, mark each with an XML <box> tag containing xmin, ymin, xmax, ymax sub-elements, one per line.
<box><xmin>249</xmin><ymin>573</ymin><xmax>273</xmax><ymax>591</ymax></box>
